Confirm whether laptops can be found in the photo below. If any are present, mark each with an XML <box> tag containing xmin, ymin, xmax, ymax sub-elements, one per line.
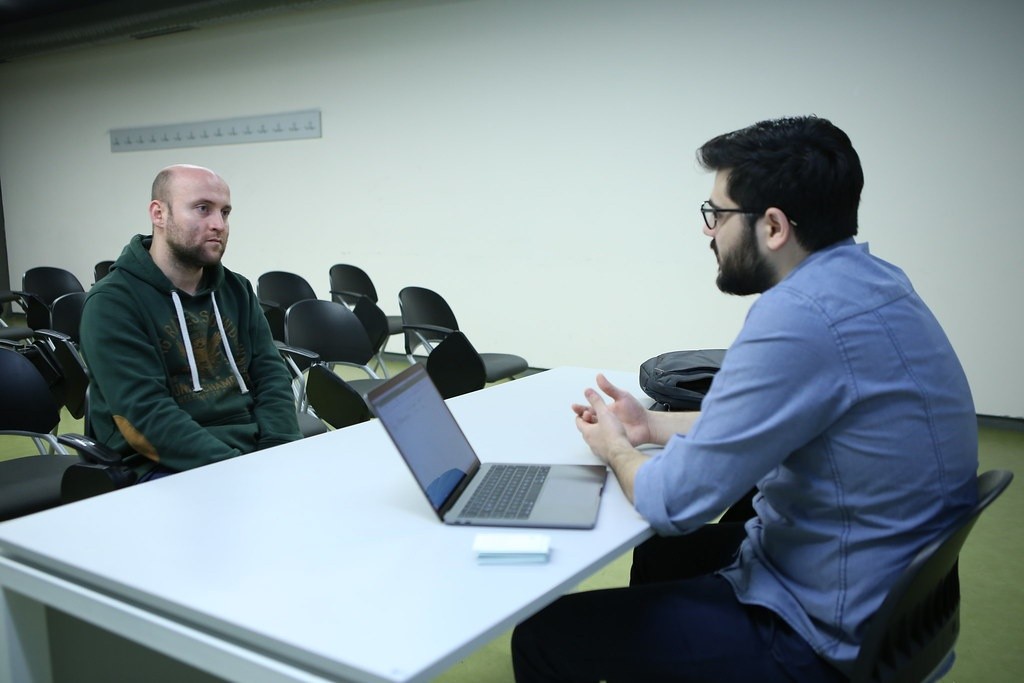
<box><xmin>364</xmin><ymin>362</ymin><xmax>607</xmax><ymax>529</ymax></box>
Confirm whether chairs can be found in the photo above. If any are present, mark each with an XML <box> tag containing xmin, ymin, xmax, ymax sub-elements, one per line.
<box><xmin>257</xmin><ymin>264</ymin><xmax>528</xmax><ymax>439</ymax></box>
<box><xmin>851</xmin><ymin>468</ymin><xmax>1016</xmax><ymax>683</ymax></box>
<box><xmin>0</xmin><ymin>260</ymin><xmax>137</xmax><ymax>525</ymax></box>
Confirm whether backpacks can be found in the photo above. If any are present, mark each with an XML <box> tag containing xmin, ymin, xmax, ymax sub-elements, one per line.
<box><xmin>639</xmin><ymin>349</ymin><xmax>727</xmax><ymax>412</ymax></box>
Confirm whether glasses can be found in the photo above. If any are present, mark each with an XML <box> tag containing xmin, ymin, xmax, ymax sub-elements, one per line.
<box><xmin>701</xmin><ymin>201</ymin><xmax>797</xmax><ymax>231</ymax></box>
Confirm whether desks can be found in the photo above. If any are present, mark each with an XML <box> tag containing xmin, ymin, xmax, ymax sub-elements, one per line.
<box><xmin>0</xmin><ymin>364</ymin><xmax>667</xmax><ymax>683</ymax></box>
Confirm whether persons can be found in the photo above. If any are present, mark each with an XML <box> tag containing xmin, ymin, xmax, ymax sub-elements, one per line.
<box><xmin>511</xmin><ymin>118</ymin><xmax>980</xmax><ymax>683</ymax></box>
<box><xmin>80</xmin><ymin>163</ymin><xmax>306</xmax><ymax>484</ymax></box>
